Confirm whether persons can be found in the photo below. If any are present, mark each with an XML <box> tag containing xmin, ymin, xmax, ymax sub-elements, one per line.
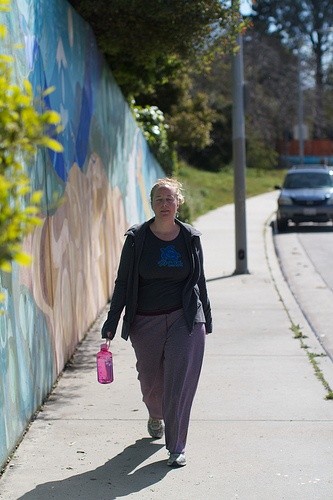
<box><xmin>100</xmin><ymin>179</ymin><xmax>213</xmax><ymax>467</ymax></box>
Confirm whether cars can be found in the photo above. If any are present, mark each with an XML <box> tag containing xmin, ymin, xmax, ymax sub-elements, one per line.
<box><xmin>273</xmin><ymin>168</ymin><xmax>333</xmax><ymax>230</ymax></box>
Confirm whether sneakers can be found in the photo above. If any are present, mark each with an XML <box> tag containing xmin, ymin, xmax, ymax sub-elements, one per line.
<box><xmin>166</xmin><ymin>453</ymin><xmax>187</xmax><ymax>466</ymax></box>
<box><xmin>147</xmin><ymin>417</ymin><xmax>163</xmax><ymax>438</ymax></box>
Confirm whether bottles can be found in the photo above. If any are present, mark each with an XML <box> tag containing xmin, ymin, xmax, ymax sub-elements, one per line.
<box><xmin>95</xmin><ymin>337</ymin><xmax>114</xmax><ymax>384</ymax></box>
